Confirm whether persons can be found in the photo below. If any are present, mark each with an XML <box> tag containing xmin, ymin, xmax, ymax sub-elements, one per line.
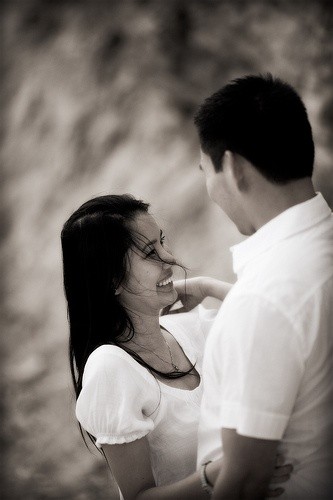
<box><xmin>60</xmin><ymin>193</ymin><xmax>294</xmax><ymax>500</ymax></box>
<box><xmin>194</xmin><ymin>69</ymin><xmax>332</xmax><ymax>500</ymax></box>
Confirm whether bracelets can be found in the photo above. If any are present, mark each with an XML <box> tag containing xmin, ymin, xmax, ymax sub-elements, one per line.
<box><xmin>200</xmin><ymin>460</ymin><xmax>214</xmax><ymax>497</ymax></box>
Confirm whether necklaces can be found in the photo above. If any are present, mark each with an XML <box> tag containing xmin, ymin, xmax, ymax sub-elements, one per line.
<box><xmin>122</xmin><ymin>329</ymin><xmax>182</xmax><ymax>372</ymax></box>
